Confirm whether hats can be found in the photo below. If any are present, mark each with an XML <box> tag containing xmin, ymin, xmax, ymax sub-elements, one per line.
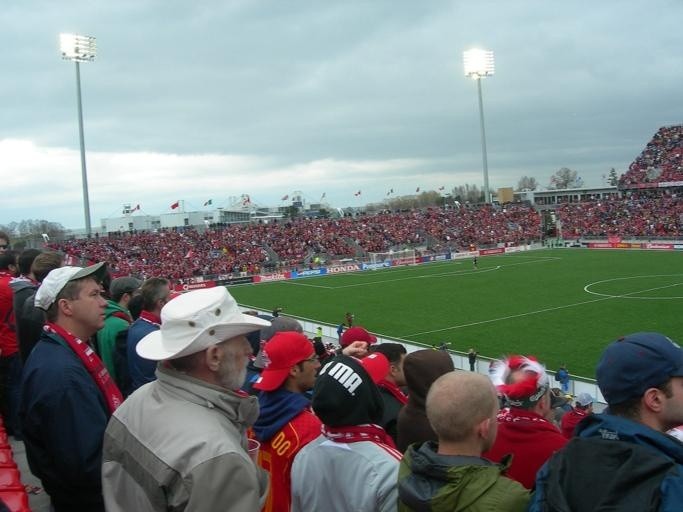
<box><xmin>134</xmin><ymin>285</ymin><xmax>272</xmax><ymax>360</ymax></box>
<box><xmin>109</xmin><ymin>277</ymin><xmax>141</xmax><ymax>295</ymax></box>
<box><xmin>575</xmin><ymin>392</ymin><xmax>594</xmax><ymax>407</ymax></box>
<box><xmin>251</xmin><ymin>331</ymin><xmax>316</xmax><ymax>391</ymax></box>
<box><xmin>32</xmin><ymin>261</ymin><xmax>104</xmax><ymax>310</ymax></box>
<box><xmin>595</xmin><ymin>333</ymin><xmax>682</xmax><ymax>404</ymax></box>
<box><xmin>340</xmin><ymin>326</ymin><xmax>377</xmax><ymax>346</ymax></box>
<box><xmin>347</xmin><ymin>351</ymin><xmax>390</xmax><ymax>384</ymax></box>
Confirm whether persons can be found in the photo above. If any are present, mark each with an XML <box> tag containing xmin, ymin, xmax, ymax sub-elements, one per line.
<box><xmin>1</xmin><ymin>124</ymin><xmax>682</xmax><ymax>512</ymax></box>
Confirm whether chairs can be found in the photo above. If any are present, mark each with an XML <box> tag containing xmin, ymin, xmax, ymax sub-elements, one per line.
<box><xmin>0</xmin><ymin>416</ymin><xmax>33</xmax><ymax>512</ymax></box>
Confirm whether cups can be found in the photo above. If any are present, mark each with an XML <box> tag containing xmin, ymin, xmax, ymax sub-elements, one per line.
<box><xmin>246</xmin><ymin>440</ymin><xmax>260</xmax><ymax>464</ymax></box>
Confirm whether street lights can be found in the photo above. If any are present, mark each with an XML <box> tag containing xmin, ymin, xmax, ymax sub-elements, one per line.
<box><xmin>461</xmin><ymin>45</ymin><xmax>496</xmax><ymax>206</ymax></box>
<box><xmin>57</xmin><ymin>31</ymin><xmax>99</xmax><ymax>240</ymax></box>
